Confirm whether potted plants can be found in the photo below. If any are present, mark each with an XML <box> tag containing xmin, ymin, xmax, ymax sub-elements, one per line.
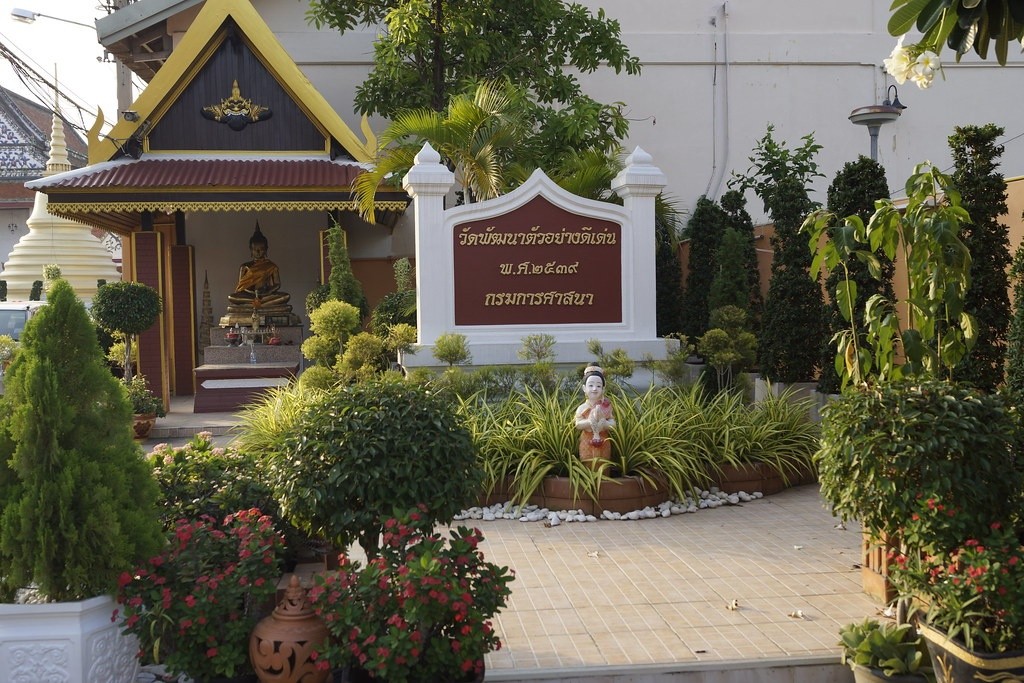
<box><xmin>811</xmin><ymin>370</ymin><xmax>1024</xmax><ymax>606</ymax></box>
<box><xmin>836</xmin><ymin>613</ymin><xmax>926</xmax><ymax>683</ymax></box>
<box><xmin>125</xmin><ymin>372</ymin><xmax>167</xmax><ymax>444</ymax></box>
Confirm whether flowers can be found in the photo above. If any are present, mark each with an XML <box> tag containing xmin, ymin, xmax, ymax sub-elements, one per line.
<box><xmin>107</xmin><ymin>507</ymin><xmax>290</xmax><ymax>683</ymax></box>
<box><xmin>308</xmin><ymin>503</ymin><xmax>518</xmax><ymax>683</ymax></box>
<box><xmin>858</xmin><ymin>486</ymin><xmax>1024</xmax><ymax>658</ymax></box>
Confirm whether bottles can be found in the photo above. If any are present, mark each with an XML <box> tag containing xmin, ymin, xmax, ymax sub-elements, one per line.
<box><xmin>250</xmin><ymin>344</ymin><xmax>256</xmax><ymax>364</ymax></box>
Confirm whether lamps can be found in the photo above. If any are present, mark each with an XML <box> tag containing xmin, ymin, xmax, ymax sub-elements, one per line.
<box><xmin>10</xmin><ymin>8</ymin><xmax>96</xmax><ymax>31</ymax></box>
<box><xmin>883</xmin><ymin>85</ymin><xmax>908</xmax><ymax>110</ymax></box>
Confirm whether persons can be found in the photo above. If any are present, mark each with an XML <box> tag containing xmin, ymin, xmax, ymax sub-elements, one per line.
<box><xmin>251</xmin><ymin>308</ymin><xmax>260</xmax><ymax>332</ymax></box>
<box><xmin>227</xmin><ymin>217</ymin><xmax>291</xmax><ymax>306</ymax></box>
<box><xmin>572</xmin><ymin>360</ymin><xmax>616</xmax><ymax>477</ymax></box>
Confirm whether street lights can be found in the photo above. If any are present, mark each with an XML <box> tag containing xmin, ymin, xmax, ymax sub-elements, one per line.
<box><xmin>11</xmin><ymin>9</ymin><xmax>135</xmax><ymax>126</ymax></box>
<box><xmin>847</xmin><ymin>85</ymin><xmax>908</xmax><ymax>162</ymax></box>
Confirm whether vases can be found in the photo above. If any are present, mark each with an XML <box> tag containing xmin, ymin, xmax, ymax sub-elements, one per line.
<box><xmin>915</xmin><ymin>613</ymin><xmax>1024</xmax><ymax>683</ymax></box>
